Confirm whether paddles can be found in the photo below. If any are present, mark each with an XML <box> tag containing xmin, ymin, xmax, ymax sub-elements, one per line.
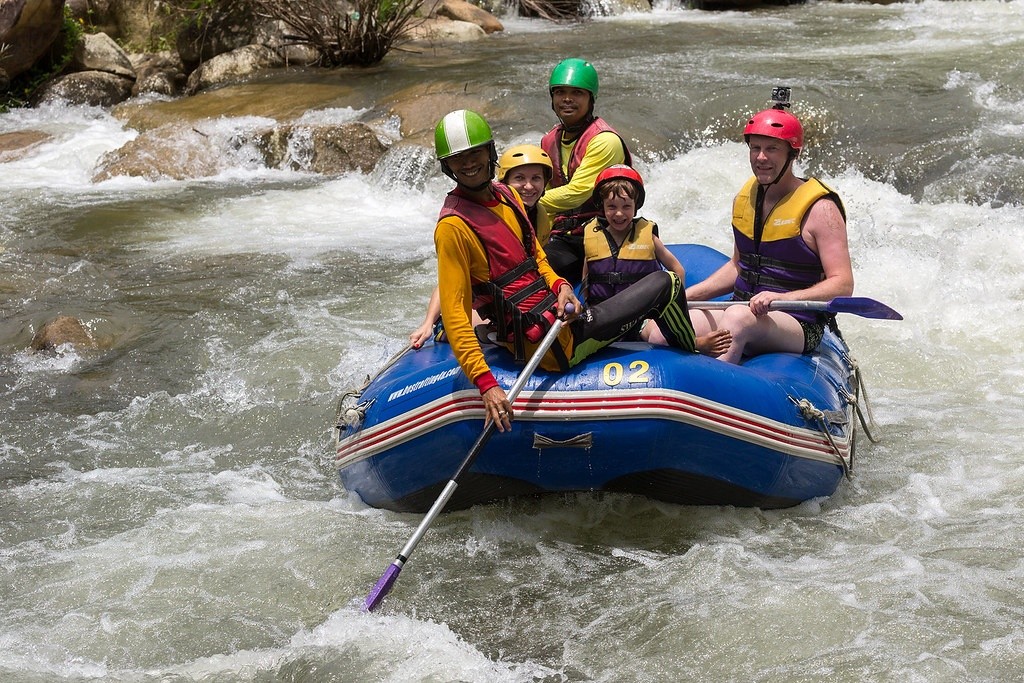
<box><xmin>369</xmin><ymin>313</ymin><xmax>570</xmax><ymax>614</ymax></box>
<box><xmin>686</xmin><ymin>293</ymin><xmax>902</xmax><ymax>320</ymax></box>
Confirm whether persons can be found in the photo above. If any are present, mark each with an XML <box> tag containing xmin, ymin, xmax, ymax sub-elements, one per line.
<box><xmin>647</xmin><ymin>109</ymin><xmax>854</xmax><ymax>364</ymax></box>
<box><xmin>410</xmin><ymin>58</ymin><xmax>731</xmax><ymax>432</ymax></box>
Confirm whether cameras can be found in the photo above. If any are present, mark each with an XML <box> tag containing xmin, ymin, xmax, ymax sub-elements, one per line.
<box><xmin>771</xmin><ymin>86</ymin><xmax>791</xmax><ymax>101</ymax></box>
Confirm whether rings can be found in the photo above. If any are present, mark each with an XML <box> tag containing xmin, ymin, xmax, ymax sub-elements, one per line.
<box><xmin>499</xmin><ymin>411</ymin><xmax>505</xmax><ymax>415</ymax></box>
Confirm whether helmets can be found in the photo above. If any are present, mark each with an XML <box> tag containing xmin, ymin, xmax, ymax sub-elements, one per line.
<box><xmin>498</xmin><ymin>143</ymin><xmax>554</xmax><ymax>183</ymax></box>
<box><xmin>592</xmin><ymin>164</ymin><xmax>645</xmax><ymax>210</ymax></box>
<box><xmin>434</xmin><ymin>108</ymin><xmax>493</xmax><ymax>160</ymax></box>
<box><xmin>549</xmin><ymin>58</ymin><xmax>598</xmax><ymax>104</ymax></box>
<box><xmin>743</xmin><ymin>108</ymin><xmax>805</xmax><ymax>159</ymax></box>
